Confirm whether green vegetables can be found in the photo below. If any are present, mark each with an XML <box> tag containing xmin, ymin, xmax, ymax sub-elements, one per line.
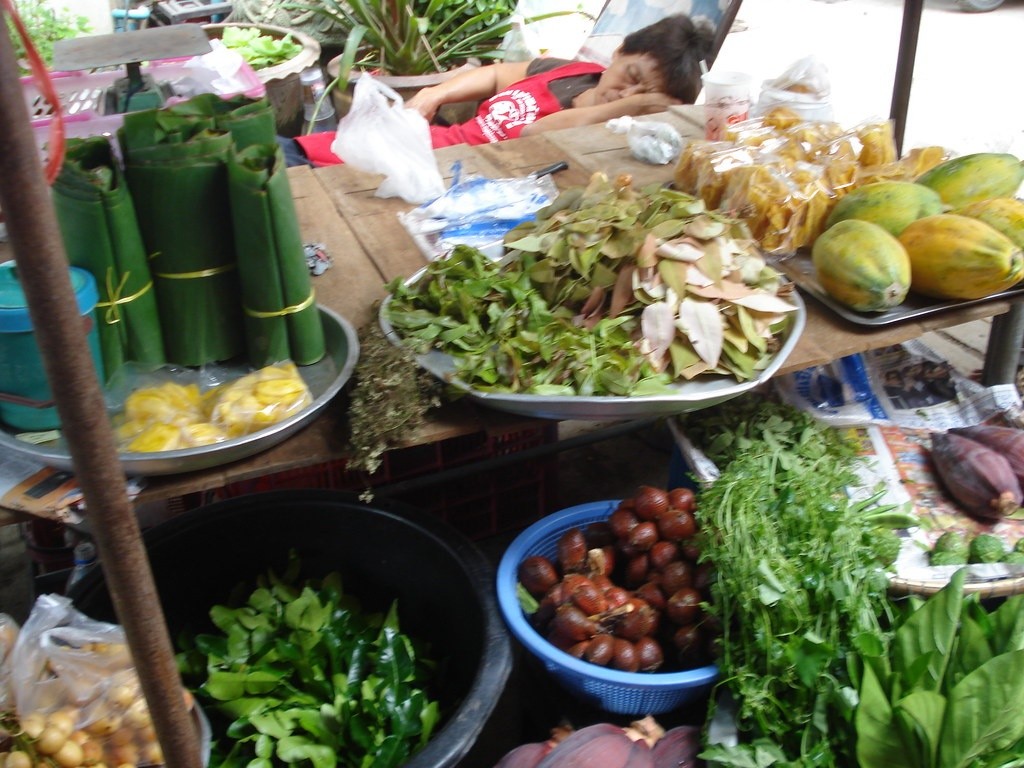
<box><xmin>171</xmin><ymin>573</ymin><xmax>443</xmax><ymax>767</ymax></box>
<box><xmin>709</xmin><ymin>451</ymin><xmax>1024</xmax><ymax>768</ymax></box>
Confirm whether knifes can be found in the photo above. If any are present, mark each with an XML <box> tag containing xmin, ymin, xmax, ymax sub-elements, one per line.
<box><xmin>424</xmin><ymin>160</ymin><xmax>569</xmax><ymax>217</ymax></box>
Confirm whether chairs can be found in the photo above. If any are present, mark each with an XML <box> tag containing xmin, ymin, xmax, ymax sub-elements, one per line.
<box><xmin>430</xmin><ymin>0</ymin><xmax>743</xmax><ymax>126</ymax></box>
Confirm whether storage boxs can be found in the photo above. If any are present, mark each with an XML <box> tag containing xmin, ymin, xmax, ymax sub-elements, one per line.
<box><xmin>209</xmin><ymin>420</ymin><xmax>560</xmax><ymax>545</ymax></box>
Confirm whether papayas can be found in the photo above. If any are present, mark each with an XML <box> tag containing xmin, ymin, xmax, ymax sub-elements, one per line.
<box><xmin>809</xmin><ymin>153</ymin><xmax>1024</xmax><ymax>314</ymax></box>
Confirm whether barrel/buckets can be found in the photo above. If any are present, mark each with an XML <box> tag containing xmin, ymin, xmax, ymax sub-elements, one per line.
<box><xmin>0</xmin><ymin>260</ymin><xmax>107</xmax><ymax>429</ymax></box>
<box><xmin>757</xmin><ymin>79</ymin><xmax>834</xmax><ymax>122</ymax></box>
<box><xmin>66</xmin><ymin>487</ymin><xmax>514</xmax><ymax>768</ymax></box>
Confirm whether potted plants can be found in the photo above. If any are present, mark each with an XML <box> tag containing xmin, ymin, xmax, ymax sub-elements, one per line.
<box><xmin>139</xmin><ymin>22</ymin><xmax>321</xmax><ymax>139</ymax></box>
<box><xmin>281</xmin><ymin>0</ymin><xmax>573</xmax><ymax>130</ymax></box>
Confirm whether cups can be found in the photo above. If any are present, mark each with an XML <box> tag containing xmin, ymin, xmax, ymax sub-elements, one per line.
<box><xmin>703</xmin><ymin>75</ymin><xmax>748</xmax><ymax>142</ymax></box>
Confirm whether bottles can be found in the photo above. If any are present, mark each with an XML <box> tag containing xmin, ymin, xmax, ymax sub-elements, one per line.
<box><xmin>67</xmin><ymin>544</ymin><xmax>97</xmax><ymax>585</ymax></box>
<box><xmin>302</xmin><ymin>67</ymin><xmax>338</xmax><ymax>134</ymax></box>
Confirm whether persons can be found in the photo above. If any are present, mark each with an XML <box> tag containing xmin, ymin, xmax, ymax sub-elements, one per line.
<box><xmin>276</xmin><ymin>14</ymin><xmax>716</xmax><ymax>168</ymax></box>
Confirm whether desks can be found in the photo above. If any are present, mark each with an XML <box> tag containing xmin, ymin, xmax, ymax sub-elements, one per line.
<box><xmin>0</xmin><ymin>100</ymin><xmax>1024</xmax><ymax>616</ymax></box>
